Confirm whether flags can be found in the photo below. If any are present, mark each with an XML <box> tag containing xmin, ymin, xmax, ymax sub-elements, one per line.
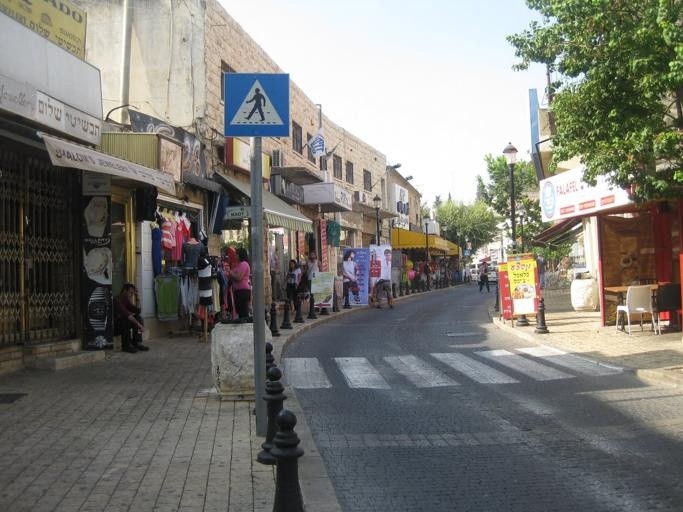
<box><xmin>307</xmin><ymin>127</ymin><xmax>326</xmax><ymax>158</ymax></box>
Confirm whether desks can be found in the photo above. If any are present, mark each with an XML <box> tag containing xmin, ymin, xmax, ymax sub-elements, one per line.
<box><xmin>604</xmin><ymin>281</ymin><xmax>672</xmax><ymax>331</ymax></box>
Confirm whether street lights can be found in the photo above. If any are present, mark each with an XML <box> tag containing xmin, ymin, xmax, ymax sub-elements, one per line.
<box><xmin>372</xmin><ymin>193</ymin><xmax>382</xmax><ymax>245</ymax></box>
<box><xmin>441</xmin><ymin>220</ymin><xmax>448</xmax><ymax>279</ymax></box>
<box><xmin>422</xmin><ymin>213</ymin><xmax>432</xmax><ymax>293</ymax></box>
<box><xmin>502</xmin><ymin>141</ymin><xmax>519</xmax><ymax>255</ymax></box>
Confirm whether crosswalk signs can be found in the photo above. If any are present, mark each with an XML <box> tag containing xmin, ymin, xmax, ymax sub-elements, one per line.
<box><xmin>223</xmin><ymin>72</ymin><xmax>289</xmax><ymax>138</ymax></box>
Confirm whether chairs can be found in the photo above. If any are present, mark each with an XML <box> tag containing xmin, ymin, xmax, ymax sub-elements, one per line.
<box><xmin>615</xmin><ymin>283</ymin><xmax>682</xmax><ymax>336</ymax></box>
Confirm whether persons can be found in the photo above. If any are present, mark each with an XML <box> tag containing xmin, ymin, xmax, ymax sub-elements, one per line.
<box><xmin>424</xmin><ymin>259</ymin><xmax>432</xmax><ymax>291</ymax></box>
<box><xmin>343</xmin><ymin>251</ymin><xmax>360</xmax><ymax>305</ymax></box>
<box><xmin>479</xmin><ymin>261</ymin><xmax>490</xmax><ymax>293</ymax></box>
<box><xmin>374</xmin><ymin>249</ymin><xmax>391</xmax><ymax>284</ymax></box>
<box><xmin>282</xmin><ymin>252</ymin><xmax>323</xmax><ymax>313</ymax></box>
<box><xmin>113</xmin><ymin>283</ymin><xmax>149</xmax><ymax>353</ymax></box>
<box><xmin>225</xmin><ymin>248</ymin><xmax>252</xmax><ymax>318</ymax></box>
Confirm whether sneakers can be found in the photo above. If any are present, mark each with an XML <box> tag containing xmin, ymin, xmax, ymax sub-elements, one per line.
<box><xmin>120</xmin><ymin>341</ymin><xmax>149</xmax><ymax>353</ymax></box>
